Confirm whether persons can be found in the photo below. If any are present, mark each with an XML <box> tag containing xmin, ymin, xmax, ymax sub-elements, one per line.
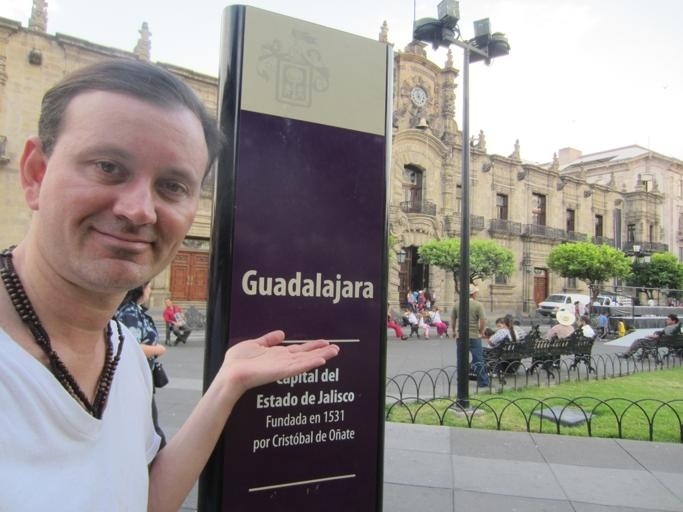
<box><xmin>114</xmin><ymin>281</ymin><xmax>166</xmax><ymax>451</ymax></box>
<box><xmin>163</xmin><ymin>298</ymin><xmax>191</xmax><ymax>345</ymax></box>
<box><xmin>387</xmin><ymin>283</ymin><xmax>683</xmax><ymax>387</ymax></box>
<box><xmin>0</xmin><ymin>58</ymin><xmax>341</xmax><ymax>509</ymax></box>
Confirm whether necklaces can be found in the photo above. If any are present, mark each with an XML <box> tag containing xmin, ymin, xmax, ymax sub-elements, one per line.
<box><xmin>1</xmin><ymin>244</ymin><xmax>124</xmax><ymax>418</ymax></box>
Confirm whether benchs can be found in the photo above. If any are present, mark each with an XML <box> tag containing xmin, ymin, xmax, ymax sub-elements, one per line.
<box><xmin>637</xmin><ymin>322</ymin><xmax>682</xmax><ymax>366</ymax></box>
<box><xmin>480</xmin><ymin>325</ymin><xmax>599</xmax><ymax>375</ymax></box>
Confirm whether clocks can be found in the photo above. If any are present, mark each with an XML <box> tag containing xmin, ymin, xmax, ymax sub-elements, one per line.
<box><xmin>411</xmin><ymin>86</ymin><xmax>428</xmax><ymax>107</ymax></box>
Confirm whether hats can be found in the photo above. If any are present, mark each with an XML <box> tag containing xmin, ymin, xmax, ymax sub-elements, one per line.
<box><xmin>469</xmin><ymin>283</ymin><xmax>479</xmax><ymax>294</ymax></box>
<box><xmin>556</xmin><ymin>311</ymin><xmax>575</xmax><ymax>326</ymax></box>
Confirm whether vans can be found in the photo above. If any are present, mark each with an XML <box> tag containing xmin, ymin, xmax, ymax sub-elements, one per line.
<box><xmin>535</xmin><ymin>293</ymin><xmax>590</xmax><ymax>318</ymax></box>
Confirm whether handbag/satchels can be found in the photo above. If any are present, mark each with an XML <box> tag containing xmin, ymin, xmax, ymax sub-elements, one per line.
<box><xmin>152</xmin><ymin>363</ymin><xmax>169</xmax><ymax>387</ymax></box>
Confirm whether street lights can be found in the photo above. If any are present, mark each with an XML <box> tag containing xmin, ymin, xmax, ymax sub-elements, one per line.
<box><xmin>632</xmin><ymin>244</ymin><xmax>640</xmax><ymax>285</ymax></box>
<box><xmin>413</xmin><ymin>0</ymin><xmax>511</xmax><ymax>408</ymax></box>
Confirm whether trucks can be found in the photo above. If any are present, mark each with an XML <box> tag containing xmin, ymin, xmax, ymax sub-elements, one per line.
<box><xmin>590</xmin><ymin>295</ymin><xmax>631</xmax><ymax>307</ymax></box>
